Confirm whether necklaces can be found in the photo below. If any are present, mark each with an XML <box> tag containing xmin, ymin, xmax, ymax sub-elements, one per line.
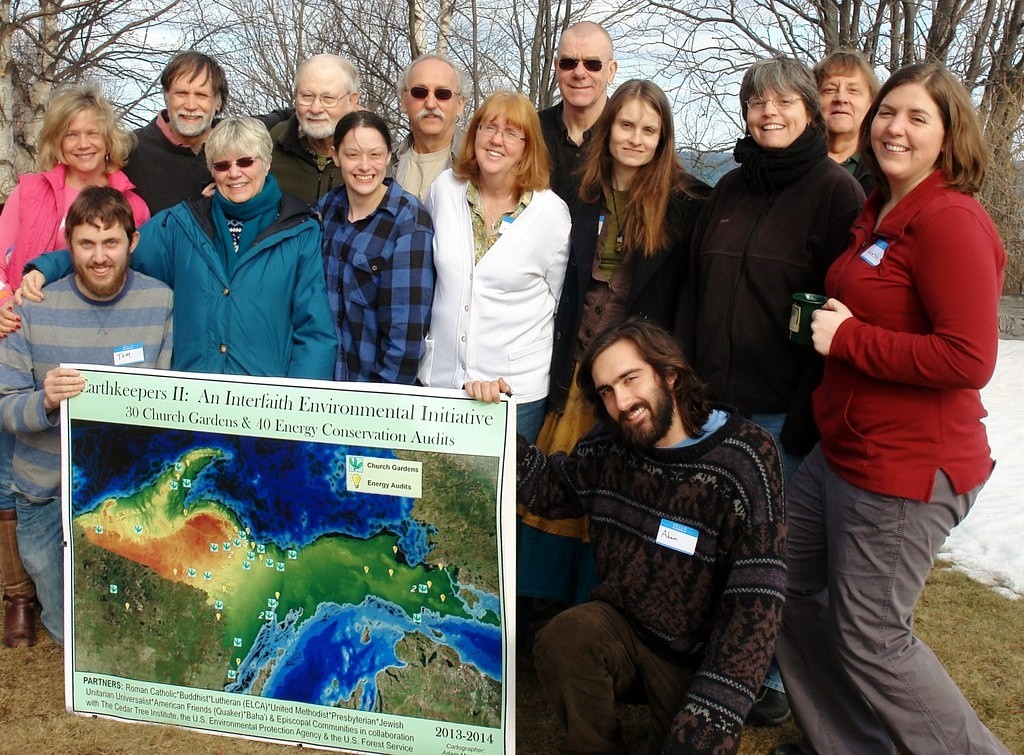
<box><xmin>478</xmin><ymin>177</ymin><xmax>518</xmax><ymax>229</ymax></box>
<box><xmin>606</xmin><ymin>169</ymin><xmax>637</xmax><ymax>253</ymax></box>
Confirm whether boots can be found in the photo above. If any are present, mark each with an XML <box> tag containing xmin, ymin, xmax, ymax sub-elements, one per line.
<box><xmin>0</xmin><ymin>508</ymin><xmax>37</xmax><ymax>645</ymax></box>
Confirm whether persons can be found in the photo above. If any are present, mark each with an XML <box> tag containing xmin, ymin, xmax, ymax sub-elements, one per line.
<box><xmin>116</xmin><ymin>21</ymin><xmax>871</xmax><ymax>459</ymax></box>
<box><xmin>775</xmin><ymin>63</ymin><xmax>1014</xmax><ymax>755</ymax></box>
<box><xmin>14</xmin><ymin>116</ymin><xmax>339</xmax><ymax>383</ymax></box>
<box><xmin>414</xmin><ymin>92</ymin><xmax>572</xmax><ymax>679</ymax></box>
<box><xmin>462</xmin><ymin>320</ymin><xmax>788</xmax><ymax>755</ymax></box>
<box><xmin>516</xmin><ymin>81</ymin><xmax>716</xmax><ymax>606</ymax></box>
<box><xmin>202</xmin><ymin>111</ymin><xmax>437</xmax><ymax>387</ymax></box>
<box><xmin>0</xmin><ymin>86</ymin><xmax>150</xmax><ymax>647</ymax></box>
<box><xmin>0</xmin><ymin>186</ymin><xmax>174</xmax><ymax>646</ymax></box>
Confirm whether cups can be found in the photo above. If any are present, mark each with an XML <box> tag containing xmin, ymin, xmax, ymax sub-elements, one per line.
<box><xmin>788</xmin><ymin>294</ymin><xmax>830</xmax><ymax>348</ymax></box>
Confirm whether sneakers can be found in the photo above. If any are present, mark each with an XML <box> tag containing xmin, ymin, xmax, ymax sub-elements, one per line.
<box><xmin>749</xmin><ymin>686</ymin><xmax>792</xmax><ymax>725</ymax></box>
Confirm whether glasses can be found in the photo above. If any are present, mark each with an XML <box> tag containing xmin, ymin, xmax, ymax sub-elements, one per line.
<box><xmin>295</xmin><ymin>91</ymin><xmax>350</xmax><ymax>108</ymax></box>
<box><xmin>476</xmin><ymin>122</ymin><xmax>527</xmax><ymax>143</ymax></box>
<box><xmin>556</xmin><ymin>57</ymin><xmax>612</xmax><ymax>72</ymax></box>
<box><xmin>212</xmin><ymin>155</ymin><xmax>259</xmax><ymax>172</ymax></box>
<box><xmin>404</xmin><ymin>87</ymin><xmax>460</xmax><ymax>100</ymax></box>
<box><xmin>744</xmin><ymin>95</ymin><xmax>804</xmax><ymax>112</ymax></box>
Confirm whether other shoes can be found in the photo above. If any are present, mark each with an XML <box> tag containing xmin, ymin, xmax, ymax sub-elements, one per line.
<box><xmin>769</xmin><ymin>743</ymin><xmax>808</xmax><ymax>755</ymax></box>
